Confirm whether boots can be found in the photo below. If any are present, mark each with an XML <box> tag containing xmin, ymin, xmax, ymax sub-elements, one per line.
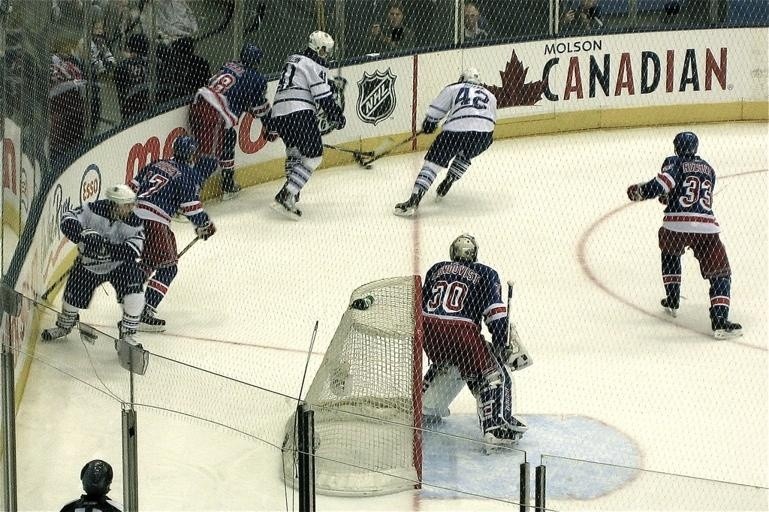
<box><xmin>395</xmin><ymin>189</ymin><xmax>425</xmax><ymax>211</ymax></box>
<box><xmin>710</xmin><ymin>305</ymin><xmax>742</xmax><ymax>331</ymax></box>
<box><xmin>117</xmin><ymin>320</ymin><xmax>143</xmax><ymax>349</ymax></box>
<box><xmin>275</xmin><ymin>180</ymin><xmax>302</xmax><ymax>216</ymax></box>
<box><xmin>483</xmin><ymin>417</ymin><xmax>522</xmax><ymax>440</ymax></box>
<box><xmin>221</xmin><ymin>169</ymin><xmax>241</xmax><ymax>193</ymax></box>
<box><xmin>436</xmin><ymin>175</ymin><xmax>454</xmax><ymax>196</ymax></box>
<box><xmin>40</xmin><ymin>310</ymin><xmax>79</xmax><ymax>341</ymax></box>
<box><xmin>661</xmin><ymin>283</ymin><xmax>680</xmax><ymax>309</ymax></box>
<box><xmin>139</xmin><ymin>305</ymin><xmax>165</xmax><ymax>325</ymax></box>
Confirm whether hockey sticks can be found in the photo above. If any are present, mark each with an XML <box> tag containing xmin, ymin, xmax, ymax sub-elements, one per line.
<box><xmin>324</xmin><ymin>137</ymin><xmax>396</xmax><ymax>154</ymax></box>
<box><xmin>355</xmin><ymin>129</ymin><xmax>425</xmax><ymax>168</ymax></box>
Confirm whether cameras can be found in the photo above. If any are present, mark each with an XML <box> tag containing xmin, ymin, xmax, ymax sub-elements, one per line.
<box><xmin>571</xmin><ymin>4</ymin><xmax>603</xmax><ymax>19</ymax></box>
<box><xmin>380</xmin><ymin>23</ymin><xmax>404</xmax><ymax>41</ymax></box>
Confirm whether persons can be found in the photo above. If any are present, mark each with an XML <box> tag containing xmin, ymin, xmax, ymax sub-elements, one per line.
<box><xmin>117</xmin><ymin>134</ymin><xmax>217</xmax><ymax>327</ymax></box>
<box><xmin>270</xmin><ymin>29</ymin><xmax>346</xmax><ymax>219</ymax></box>
<box><xmin>0</xmin><ymin>0</ymin><xmax>212</xmax><ymax>156</ymax></box>
<box><xmin>628</xmin><ymin>130</ymin><xmax>744</xmax><ymax>330</ymax></box>
<box><xmin>393</xmin><ymin>66</ymin><xmax>498</xmax><ymax>211</ymax></box>
<box><xmin>190</xmin><ymin>45</ymin><xmax>277</xmax><ymax>195</ymax></box>
<box><xmin>360</xmin><ymin>2</ymin><xmax>417</xmax><ymax>54</ymax></box>
<box><xmin>60</xmin><ymin>458</ymin><xmax>122</xmax><ymax>512</ymax></box>
<box><xmin>419</xmin><ymin>232</ymin><xmax>532</xmax><ymax>446</ymax></box>
<box><xmin>448</xmin><ymin>3</ymin><xmax>492</xmax><ymax>42</ymax></box>
<box><xmin>40</xmin><ymin>183</ymin><xmax>146</xmax><ymax>349</ymax></box>
<box><xmin>558</xmin><ymin>0</ymin><xmax>602</xmax><ymax>30</ymax></box>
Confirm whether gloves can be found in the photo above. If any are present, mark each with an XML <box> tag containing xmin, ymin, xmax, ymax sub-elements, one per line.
<box><xmin>264</xmin><ymin>124</ymin><xmax>278</xmax><ymax>142</ymax></box>
<box><xmin>195</xmin><ymin>218</ymin><xmax>215</xmax><ymax>240</ymax></box>
<box><xmin>326</xmin><ymin>113</ymin><xmax>346</xmax><ymax>130</ymax></box>
<box><xmin>658</xmin><ymin>195</ymin><xmax>668</xmax><ymax>205</ymax></box>
<box><xmin>627</xmin><ymin>184</ymin><xmax>646</xmax><ymax>201</ymax></box>
<box><xmin>423</xmin><ymin>119</ymin><xmax>438</xmax><ymax>134</ymax></box>
<box><xmin>77</xmin><ymin>227</ymin><xmax>111</xmax><ymax>262</ymax></box>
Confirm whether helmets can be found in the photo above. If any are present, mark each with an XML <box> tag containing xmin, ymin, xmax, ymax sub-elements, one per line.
<box><xmin>672</xmin><ymin>131</ymin><xmax>698</xmax><ymax>156</ymax></box>
<box><xmin>81</xmin><ymin>461</ymin><xmax>113</xmax><ymax>496</ymax></box>
<box><xmin>173</xmin><ymin>134</ymin><xmax>198</xmax><ymax>159</ymax></box>
<box><xmin>308</xmin><ymin>30</ymin><xmax>334</xmax><ymax>59</ymax></box>
<box><xmin>458</xmin><ymin>68</ymin><xmax>480</xmax><ymax>85</ymax></box>
<box><xmin>240</xmin><ymin>44</ymin><xmax>263</xmax><ymax>66</ymax></box>
<box><xmin>106</xmin><ymin>184</ymin><xmax>136</xmax><ymax>205</ymax></box>
<box><xmin>449</xmin><ymin>233</ymin><xmax>478</xmax><ymax>262</ymax></box>
